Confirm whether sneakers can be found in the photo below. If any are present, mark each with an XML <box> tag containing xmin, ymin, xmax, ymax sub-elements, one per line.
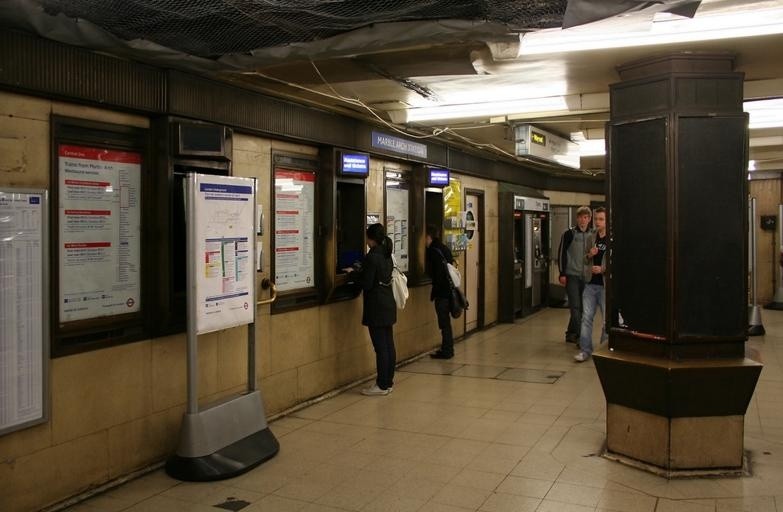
<box><xmin>429</xmin><ymin>349</ymin><xmax>455</xmax><ymax>359</ymax></box>
<box><xmin>566</xmin><ymin>335</ymin><xmax>590</xmax><ymax>362</ymax></box>
<box><xmin>360</xmin><ymin>385</ymin><xmax>392</xmax><ymax>396</ymax></box>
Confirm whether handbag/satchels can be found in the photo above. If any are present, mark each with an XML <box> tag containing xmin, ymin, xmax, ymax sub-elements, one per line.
<box><xmin>391</xmin><ymin>267</ymin><xmax>410</xmax><ymax>311</ymax></box>
<box><xmin>450</xmin><ymin>286</ymin><xmax>469</xmax><ymax>319</ymax></box>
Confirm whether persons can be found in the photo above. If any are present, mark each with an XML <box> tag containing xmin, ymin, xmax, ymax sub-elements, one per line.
<box><xmin>342</xmin><ymin>222</ymin><xmax>410</xmax><ymax>395</ymax></box>
<box><xmin>425</xmin><ymin>223</ymin><xmax>455</xmax><ymax>360</ymax></box>
<box><xmin>558</xmin><ymin>205</ymin><xmax>607</xmax><ymax>362</ymax></box>
